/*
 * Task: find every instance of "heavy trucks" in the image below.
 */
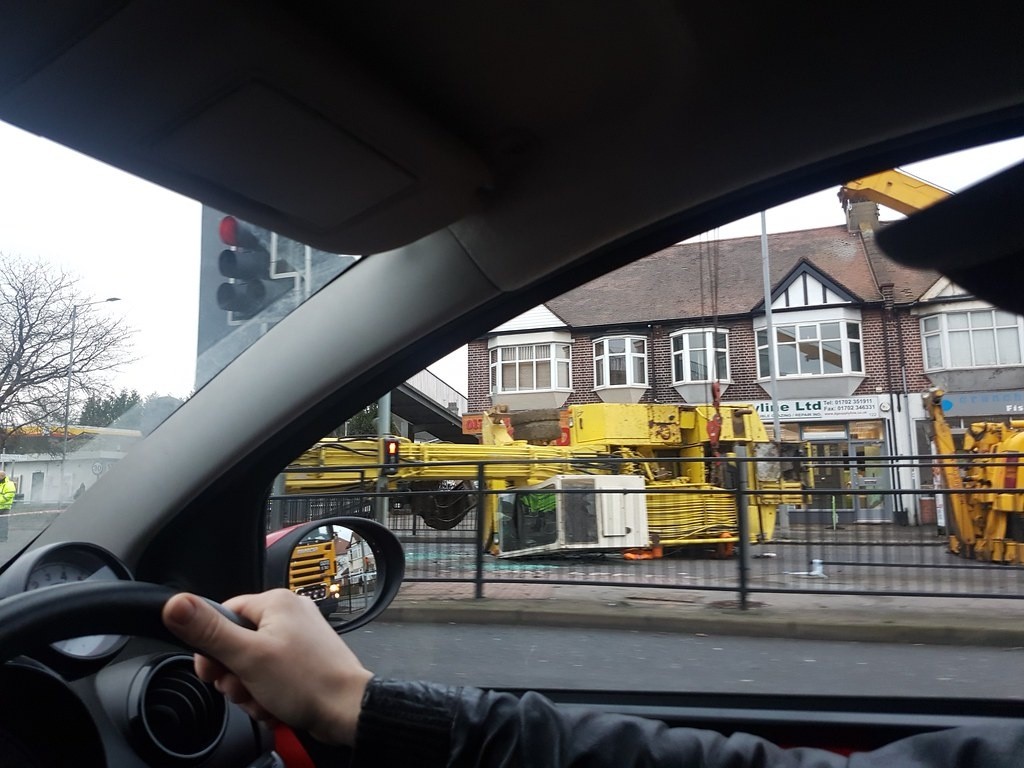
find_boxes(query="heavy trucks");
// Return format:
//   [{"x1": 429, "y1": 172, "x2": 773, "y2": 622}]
[{"x1": 287, "y1": 524, "x2": 344, "y2": 617}]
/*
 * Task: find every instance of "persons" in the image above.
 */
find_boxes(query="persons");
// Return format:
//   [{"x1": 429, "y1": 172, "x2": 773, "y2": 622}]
[
  {"x1": 0, "y1": 471, "x2": 16, "y2": 542},
  {"x1": 162, "y1": 585, "x2": 1022, "y2": 768},
  {"x1": 72, "y1": 482, "x2": 86, "y2": 500}
]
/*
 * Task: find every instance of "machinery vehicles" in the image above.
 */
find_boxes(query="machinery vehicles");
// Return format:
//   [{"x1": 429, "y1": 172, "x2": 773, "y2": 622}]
[
  {"x1": 283, "y1": 389, "x2": 782, "y2": 553},
  {"x1": 836, "y1": 160, "x2": 1023, "y2": 565}
]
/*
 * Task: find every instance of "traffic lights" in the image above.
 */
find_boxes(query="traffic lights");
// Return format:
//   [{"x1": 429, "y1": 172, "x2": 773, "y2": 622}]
[{"x1": 215, "y1": 209, "x2": 286, "y2": 324}]
